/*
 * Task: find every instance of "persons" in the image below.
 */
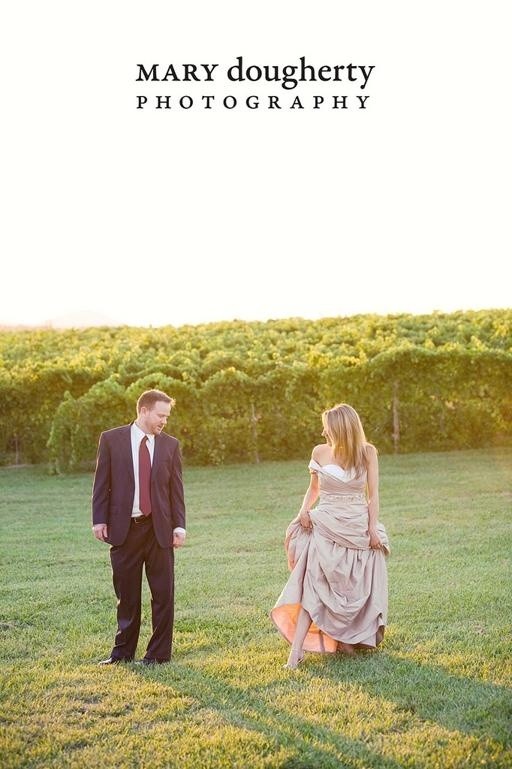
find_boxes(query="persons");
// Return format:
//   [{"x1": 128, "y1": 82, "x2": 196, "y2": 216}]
[
  {"x1": 265, "y1": 402, "x2": 391, "y2": 673},
  {"x1": 89, "y1": 389, "x2": 186, "y2": 667}
]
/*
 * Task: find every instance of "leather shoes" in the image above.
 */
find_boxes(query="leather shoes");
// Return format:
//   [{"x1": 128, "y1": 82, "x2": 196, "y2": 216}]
[
  {"x1": 99, "y1": 655, "x2": 132, "y2": 663},
  {"x1": 135, "y1": 657, "x2": 164, "y2": 665}
]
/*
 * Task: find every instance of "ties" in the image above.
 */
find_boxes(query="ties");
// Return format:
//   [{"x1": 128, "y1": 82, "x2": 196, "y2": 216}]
[{"x1": 139, "y1": 436, "x2": 152, "y2": 517}]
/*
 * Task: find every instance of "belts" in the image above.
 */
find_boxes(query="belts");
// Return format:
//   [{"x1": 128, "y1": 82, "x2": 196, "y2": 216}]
[{"x1": 132, "y1": 516, "x2": 148, "y2": 524}]
